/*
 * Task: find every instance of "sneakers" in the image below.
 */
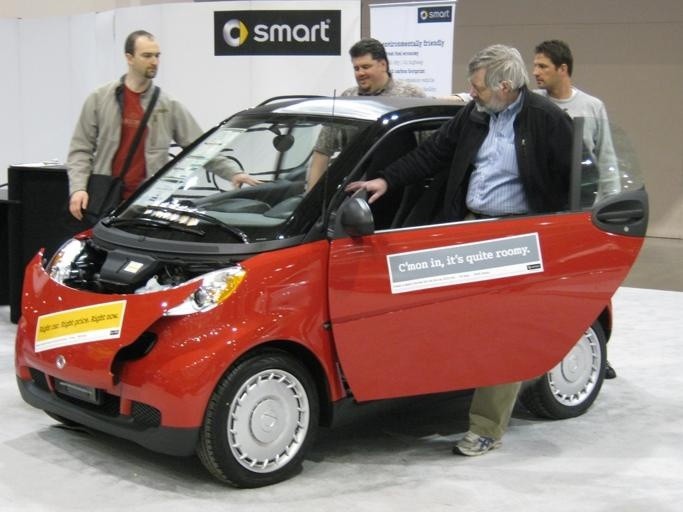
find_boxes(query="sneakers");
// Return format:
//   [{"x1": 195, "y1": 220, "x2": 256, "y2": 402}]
[{"x1": 452, "y1": 432, "x2": 502, "y2": 456}]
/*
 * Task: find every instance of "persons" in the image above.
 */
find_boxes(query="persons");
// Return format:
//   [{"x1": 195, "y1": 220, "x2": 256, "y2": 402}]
[
  {"x1": 306, "y1": 37, "x2": 435, "y2": 219},
  {"x1": 343, "y1": 44, "x2": 599, "y2": 458},
  {"x1": 429, "y1": 39, "x2": 621, "y2": 379},
  {"x1": 66, "y1": 29, "x2": 263, "y2": 232}
]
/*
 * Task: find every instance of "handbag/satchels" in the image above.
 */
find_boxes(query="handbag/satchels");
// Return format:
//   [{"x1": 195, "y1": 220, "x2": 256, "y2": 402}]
[{"x1": 61, "y1": 172, "x2": 124, "y2": 226}]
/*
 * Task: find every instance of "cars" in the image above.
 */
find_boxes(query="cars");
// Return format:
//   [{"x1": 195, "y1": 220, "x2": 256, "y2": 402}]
[{"x1": 16, "y1": 91, "x2": 648, "y2": 489}]
